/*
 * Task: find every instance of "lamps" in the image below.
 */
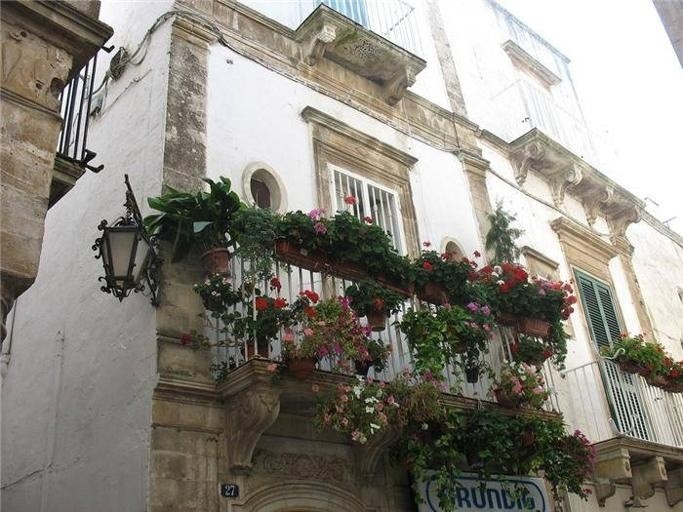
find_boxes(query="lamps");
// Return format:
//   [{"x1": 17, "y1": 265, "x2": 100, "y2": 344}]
[{"x1": 92, "y1": 174, "x2": 159, "y2": 308}]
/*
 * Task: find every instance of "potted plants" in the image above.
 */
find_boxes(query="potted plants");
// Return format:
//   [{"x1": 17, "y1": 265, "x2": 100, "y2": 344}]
[{"x1": 142, "y1": 176, "x2": 241, "y2": 281}]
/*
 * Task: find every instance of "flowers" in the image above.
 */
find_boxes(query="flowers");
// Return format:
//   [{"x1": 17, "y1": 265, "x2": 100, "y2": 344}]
[{"x1": 599, "y1": 330, "x2": 683, "y2": 394}]
